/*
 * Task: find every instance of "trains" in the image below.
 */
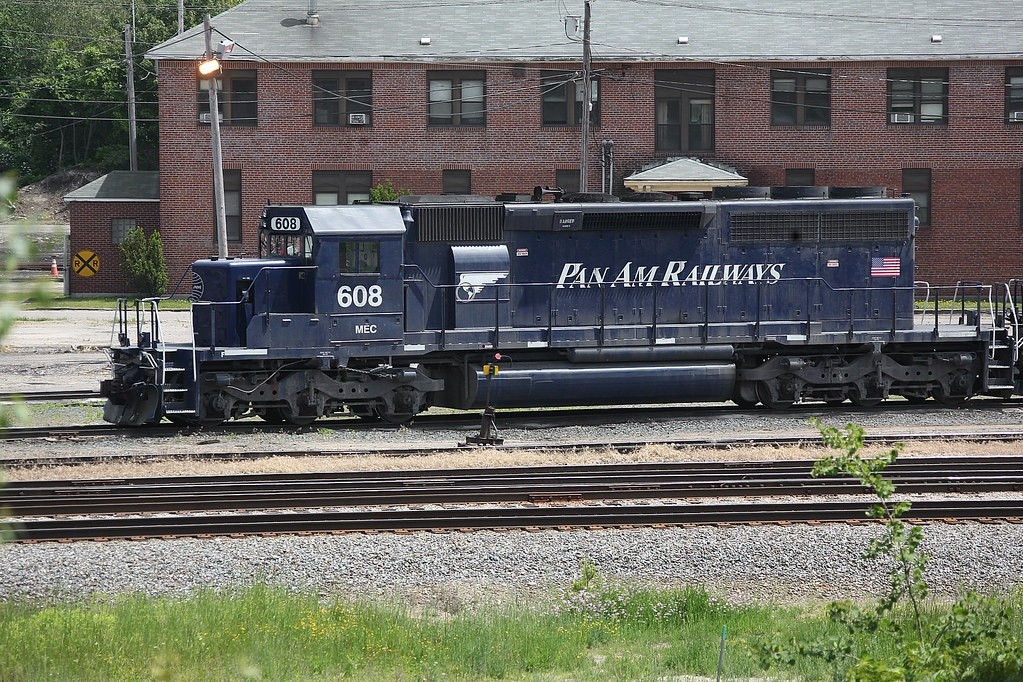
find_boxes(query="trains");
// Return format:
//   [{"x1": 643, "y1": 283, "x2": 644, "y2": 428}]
[{"x1": 100, "y1": 186, "x2": 1023, "y2": 429}]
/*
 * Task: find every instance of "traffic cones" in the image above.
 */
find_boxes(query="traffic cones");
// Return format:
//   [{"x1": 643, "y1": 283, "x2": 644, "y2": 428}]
[{"x1": 50, "y1": 258, "x2": 58, "y2": 275}]
